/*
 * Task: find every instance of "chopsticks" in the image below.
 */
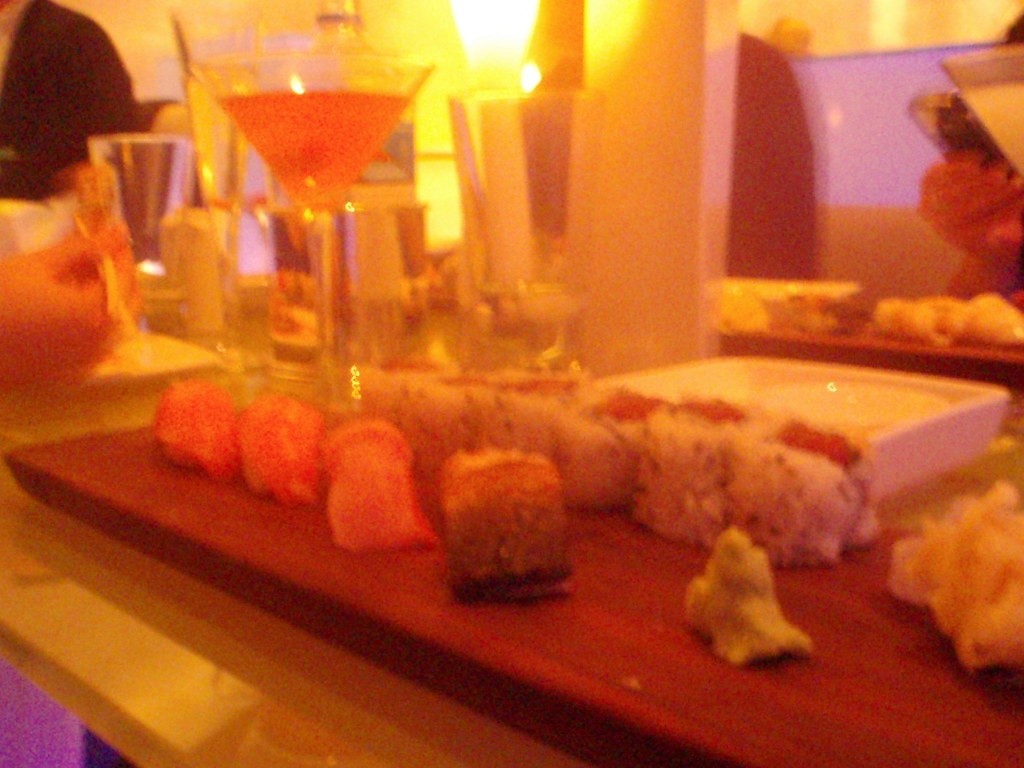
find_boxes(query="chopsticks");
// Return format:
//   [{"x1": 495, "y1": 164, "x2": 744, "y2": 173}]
[{"x1": 71, "y1": 210, "x2": 137, "y2": 338}]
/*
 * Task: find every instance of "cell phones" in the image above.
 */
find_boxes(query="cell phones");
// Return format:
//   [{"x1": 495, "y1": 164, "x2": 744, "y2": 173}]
[{"x1": 910, "y1": 91, "x2": 1018, "y2": 181}]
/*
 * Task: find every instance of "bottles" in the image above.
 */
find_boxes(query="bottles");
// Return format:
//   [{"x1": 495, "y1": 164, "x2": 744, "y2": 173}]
[{"x1": 264, "y1": 0, "x2": 429, "y2": 396}]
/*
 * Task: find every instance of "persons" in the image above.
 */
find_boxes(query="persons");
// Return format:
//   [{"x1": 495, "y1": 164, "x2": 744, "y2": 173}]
[
  {"x1": 1, "y1": 0, "x2": 145, "y2": 208},
  {"x1": 0, "y1": 165, "x2": 146, "y2": 401},
  {"x1": 917, "y1": 12, "x2": 1024, "y2": 310}
]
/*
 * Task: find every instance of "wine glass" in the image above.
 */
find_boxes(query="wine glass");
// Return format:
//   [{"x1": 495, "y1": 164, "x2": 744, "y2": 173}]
[{"x1": 189, "y1": 60, "x2": 437, "y2": 416}]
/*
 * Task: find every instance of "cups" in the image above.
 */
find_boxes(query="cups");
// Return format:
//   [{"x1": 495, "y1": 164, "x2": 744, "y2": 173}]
[
  {"x1": 450, "y1": 89, "x2": 603, "y2": 376},
  {"x1": 934, "y1": 43, "x2": 1024, "y2": 177},
  {"x1": 85, "y1": 133, "x2": 195, "y2": 347}
]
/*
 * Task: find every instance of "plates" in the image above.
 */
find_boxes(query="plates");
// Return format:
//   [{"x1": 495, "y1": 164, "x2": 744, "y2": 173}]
[{"x1": 587, "y1": 356, "x2": 1010, "y2": 493}]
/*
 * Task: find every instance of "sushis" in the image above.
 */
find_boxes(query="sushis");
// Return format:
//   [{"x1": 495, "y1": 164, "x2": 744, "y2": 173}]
[{"x1": 155, "y1": 363, "x2": 866, "y2": 591}]
